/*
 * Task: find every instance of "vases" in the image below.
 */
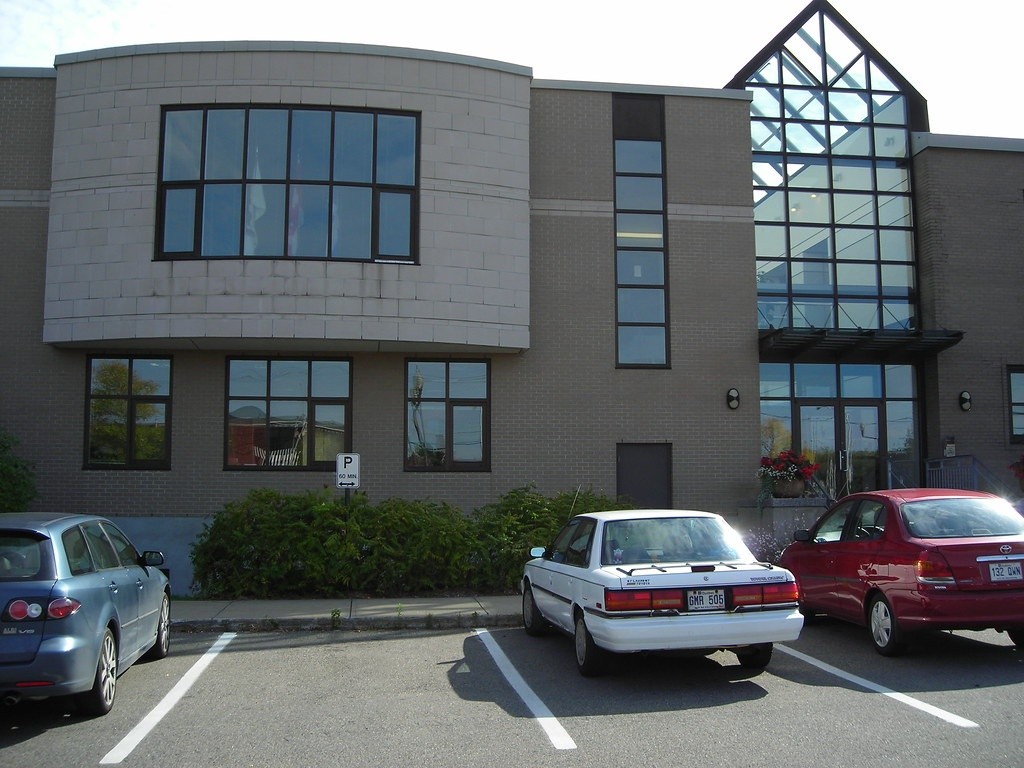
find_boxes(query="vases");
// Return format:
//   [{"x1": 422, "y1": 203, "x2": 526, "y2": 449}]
[{"x1": 772, "y1": 479, "x2": 804, "y2": 499}]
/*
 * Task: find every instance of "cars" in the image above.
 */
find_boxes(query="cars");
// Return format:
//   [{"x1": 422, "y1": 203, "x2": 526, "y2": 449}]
[
  {"x1": 776, "y1": 487, "x2": 1024, "y2": 657},
  {"x1": 0, "y1": 512, "x2": 172, "y2": 716},
  {"x1": 520, "y1": 508, "x2": 805, "y2": 677}
]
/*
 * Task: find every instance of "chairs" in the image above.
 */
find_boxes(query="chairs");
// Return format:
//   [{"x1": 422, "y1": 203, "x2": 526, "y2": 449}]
[
  {"x1": 873, "y1": 508, "x2": 884, "y2": 525},
  {"x1": 620, "y1": 544, "x2": 653, "y2": 563}
]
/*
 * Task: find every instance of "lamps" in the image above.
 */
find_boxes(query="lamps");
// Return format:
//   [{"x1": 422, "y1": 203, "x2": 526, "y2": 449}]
[
  {"x1": 727, "y1": 387, "x2": 739, "y2": 409},
  {"x1": 959, "y1": 391, "x2": 971, "y2": 411}
]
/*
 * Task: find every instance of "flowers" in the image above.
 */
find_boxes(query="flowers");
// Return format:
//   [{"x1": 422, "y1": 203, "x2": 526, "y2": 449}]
[{"x1": 754, "y1": 448, "x2": 820, "y2": 513}]
